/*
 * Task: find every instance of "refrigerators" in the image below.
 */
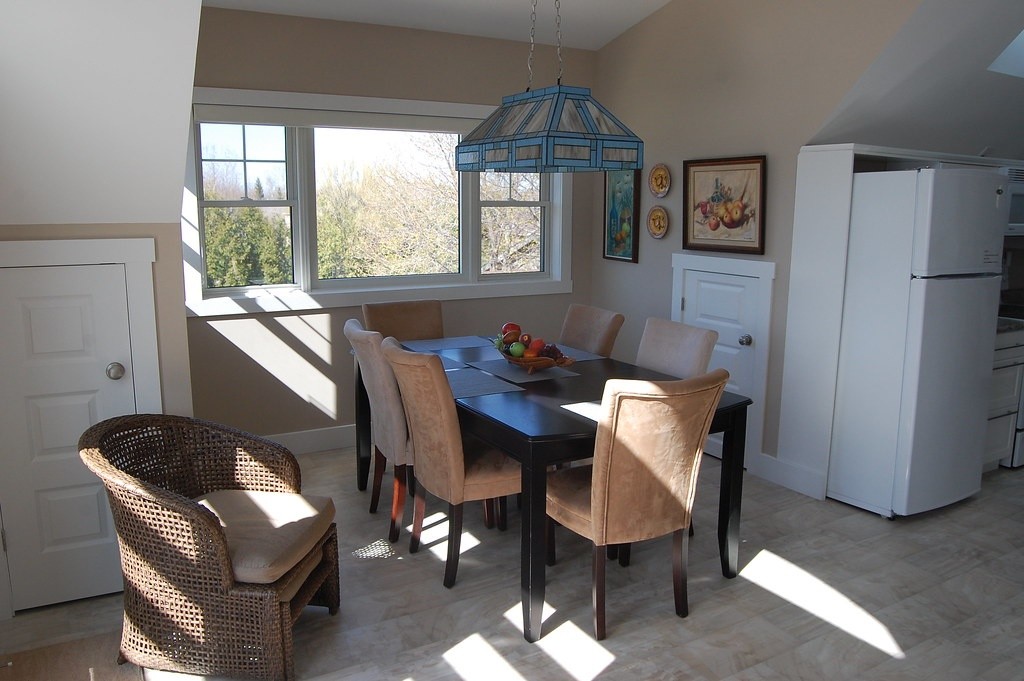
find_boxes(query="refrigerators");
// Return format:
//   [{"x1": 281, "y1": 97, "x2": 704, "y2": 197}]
[{"x1": 826, "y1": 164, "x2": 1010, "y2": 519}]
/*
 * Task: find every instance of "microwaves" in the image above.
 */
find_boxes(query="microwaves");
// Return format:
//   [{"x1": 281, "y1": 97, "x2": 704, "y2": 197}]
[{"x1": 998, "y1": 164, "x2": 1024, "y2": 238}]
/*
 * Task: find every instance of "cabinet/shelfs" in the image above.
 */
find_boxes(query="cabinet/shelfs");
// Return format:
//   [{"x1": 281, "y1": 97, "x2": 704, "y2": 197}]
[{"x1": 982, "y1": 342, "x2": 1024, "y2": 474}]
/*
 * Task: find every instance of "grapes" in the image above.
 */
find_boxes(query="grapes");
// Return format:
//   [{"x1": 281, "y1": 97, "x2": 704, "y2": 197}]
[
  {"x1": 503, "y1": 341, "x2": 513, "y2": 356},
  {"x1": 538, "y1": 343, "x2": 564, "y2": 361}
]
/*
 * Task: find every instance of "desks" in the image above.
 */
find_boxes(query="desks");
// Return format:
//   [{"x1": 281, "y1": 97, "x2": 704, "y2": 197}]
[{"x1": 398, "y1": 334, "x2": 754, "y2": 644}]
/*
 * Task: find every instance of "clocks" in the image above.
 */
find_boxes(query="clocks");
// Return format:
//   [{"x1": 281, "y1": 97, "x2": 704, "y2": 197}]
[
  {"x1": 646, "y1": 205, "x2": 670, "y2": 240},
  {"x1": 648, "y1": 163, "x2": 671, "y2": 199}
]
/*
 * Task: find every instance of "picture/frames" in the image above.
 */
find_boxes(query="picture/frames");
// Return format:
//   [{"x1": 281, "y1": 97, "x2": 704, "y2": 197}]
[
  {"x1": 602, "y1": 168, "x2": 641, "y2": 264},
  {"x1": 681, "y1": 155, "x2": 767, "y2": 255}
]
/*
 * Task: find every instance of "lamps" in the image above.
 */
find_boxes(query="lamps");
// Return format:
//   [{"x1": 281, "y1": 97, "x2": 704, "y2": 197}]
[{"x1": 453, "y1": 0, "x2": 650, "y2": 174}]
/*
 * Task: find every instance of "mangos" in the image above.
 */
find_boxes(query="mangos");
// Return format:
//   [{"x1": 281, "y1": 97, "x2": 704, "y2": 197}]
[{"x1": 501, "y1": 322, "x2": 545, "y2": 359}]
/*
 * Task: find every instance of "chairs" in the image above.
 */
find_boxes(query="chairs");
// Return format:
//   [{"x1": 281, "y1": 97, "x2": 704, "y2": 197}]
[
  {"x1": 635, "y1": 316, "x2": 720, "y2": 383},
  {"x1": 343, "y1": 318, "x2": 556, "y2": 589},
  {"x1": 544, "y1": 367, "x2": 730, "y2": 638},
  {"x1": 361, "y1": 300, "x2": 445, "y2": 340},
  {"x1": 74, "y1": 411, "x2": 343, "y2": 681},
  {"x1": 556, "y1": 303, "x2": 627, "y2": 359}
]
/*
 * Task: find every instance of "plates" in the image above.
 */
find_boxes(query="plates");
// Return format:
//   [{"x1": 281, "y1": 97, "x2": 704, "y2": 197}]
[
  {"x1": 648, "y1": 163, "x2": 671, "y2": 198},
  {"x1": 646, "y1": 205, "x2": 670, "y2": 240}
]
